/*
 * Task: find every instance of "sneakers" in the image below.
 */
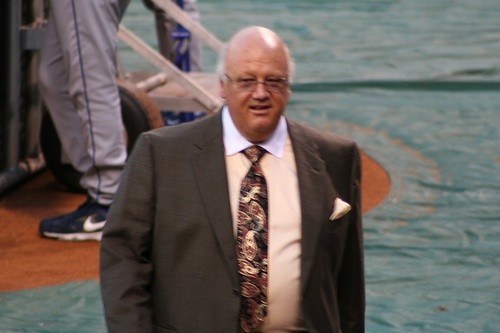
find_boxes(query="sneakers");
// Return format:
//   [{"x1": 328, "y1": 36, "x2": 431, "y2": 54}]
[{"x1": 38, "y1": 197, "x2": 110, "y2": 242}]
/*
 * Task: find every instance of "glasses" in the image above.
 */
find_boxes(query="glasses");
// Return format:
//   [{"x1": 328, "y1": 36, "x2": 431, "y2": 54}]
[{"x1": 224, "y1": 73, "x2": 286, "y2": 88}]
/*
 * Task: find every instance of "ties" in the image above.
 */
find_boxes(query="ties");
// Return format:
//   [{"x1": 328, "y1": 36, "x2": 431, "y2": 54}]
[{"x1": 236, "y1": 146, "x2": 268, "y2": 331}]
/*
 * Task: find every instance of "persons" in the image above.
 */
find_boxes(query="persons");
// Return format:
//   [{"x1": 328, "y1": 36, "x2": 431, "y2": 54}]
[
  {"x1": 35, "y1": 0, "x2": 129, "y2": 240},
  {"x1": 97, "y1": 25, "x2": 366, "y2": 333},
  {"x1": 143, "y1": 0, "x2": 213, "y2": 124}
]
